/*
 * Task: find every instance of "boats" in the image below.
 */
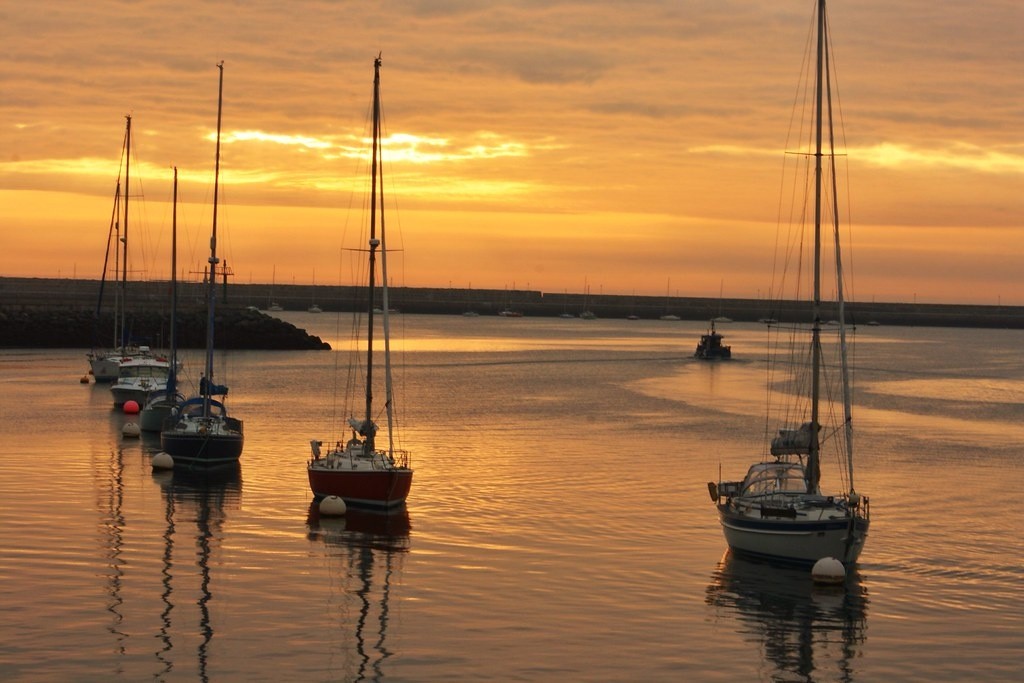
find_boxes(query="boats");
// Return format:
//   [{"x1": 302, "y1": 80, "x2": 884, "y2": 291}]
[{"x1": 694, "y1": 317, "x2": 731, "y2": 359}]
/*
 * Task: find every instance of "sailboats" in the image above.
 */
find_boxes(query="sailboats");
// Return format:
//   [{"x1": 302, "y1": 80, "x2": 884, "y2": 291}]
[
  {"x1": 709, "y1": 0, "x2": 868, "y2": 568},
  {"x1": 160, "y1": 60, "x2": 245, "y2": 478},
  {"x1": 268, "y1": 263, "x2": 282, "y2": 311},
  {"x1": 711, "y1": 278, "x2": 733, "y2": 323},
  {"x1": 308, "y1": 497, "x2": 411, "y2": 679},
  {"x1": 86, "y1": 115, "x2": 184, "y2": 432},
  {"x1": 462, "y1": 283, "x2": 479, "y2": 316},
  {"x1": 758, "y1": 289, "x2": 780, "y2": 323},
  {"x1": 308, "y1": 53, "x2": 413, "y2": 509},
  {"x1": 704, "y1": 544, "x2": 866, "y2": 683},
  {"x1": 661, "y1": 277, "x2": 681, "y2": 320},
  {"x1": 561, "y1": 281, "x2": 597, "y2": 319},
  {"x1": 499, "y1": 282, "x2": 521, "y2": 316},
  {"x1": 373, "y1": 276, "x2": 399, "y2": 314}
]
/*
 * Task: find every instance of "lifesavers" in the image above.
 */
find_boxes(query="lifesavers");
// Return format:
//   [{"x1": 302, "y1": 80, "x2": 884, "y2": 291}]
[
  {"x1": 707, "y1": 481, "x2": 718, "y2": 501},
  {"x1": 346, "y1": 439, "x2": 363, "y2": 449}
]
